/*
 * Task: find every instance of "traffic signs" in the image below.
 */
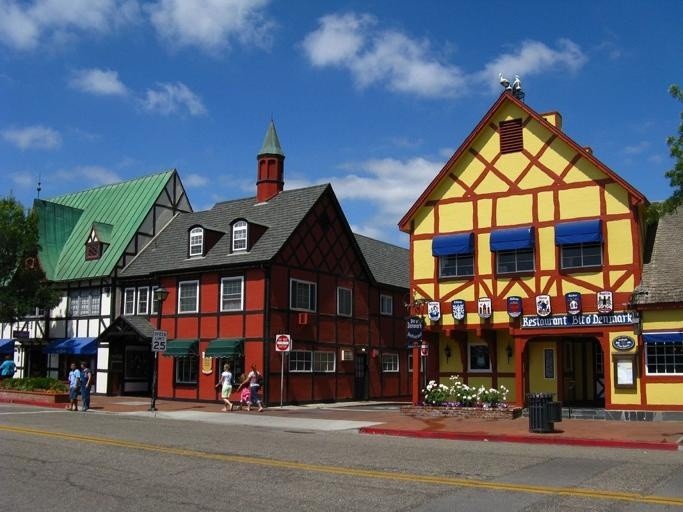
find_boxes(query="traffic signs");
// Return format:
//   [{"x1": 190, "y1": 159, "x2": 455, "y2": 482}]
[
  {"x1": 13, "y1": 331, "x2": 29, "y2": 340},
  {"x1": 150, "y1": 330, "x2": 166, "y2": 352}
]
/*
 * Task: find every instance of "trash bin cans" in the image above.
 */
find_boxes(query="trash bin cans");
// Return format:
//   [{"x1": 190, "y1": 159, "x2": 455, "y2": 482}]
[{"x1": 527, "y1": 392, "x2": 562, "y2": 433}]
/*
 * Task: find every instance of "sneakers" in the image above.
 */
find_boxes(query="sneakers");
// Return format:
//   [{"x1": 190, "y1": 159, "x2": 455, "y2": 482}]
[
  {"x1": 221, "y1": 403, "x2": 265, "y2": 412},
  {"x1": 66, "y1": 407, "x2": 90, "y2": 412}
]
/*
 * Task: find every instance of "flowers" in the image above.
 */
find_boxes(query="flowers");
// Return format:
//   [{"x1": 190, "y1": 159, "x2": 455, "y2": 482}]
[{"x1": 421, "y1": 373, "x2": 509, "y2": 407}]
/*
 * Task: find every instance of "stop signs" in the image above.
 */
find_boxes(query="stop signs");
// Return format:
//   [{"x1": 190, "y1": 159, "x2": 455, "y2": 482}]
[{"x1": 274, "y1": 334, "x2": 290, "y2": 352}]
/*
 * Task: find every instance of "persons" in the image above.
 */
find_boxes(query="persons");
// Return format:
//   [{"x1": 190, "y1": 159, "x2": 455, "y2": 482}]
[
  {"x1": 241, "y1": 362, "x2": 264, "y2": 412},
  {"x1": 215, "y1": 364, "x2": 234, "y2": 412},
  {"x1": 66, "y1": 363, "x2": 81, "y2": 411},
  {"x1": 0, "y1": 355, "x2": 17, "y2": 378},
  {"x1": 237, "y1": 374, "x2": 253, "y2": 411},
  {"x1": 78, "y1": 361, "x2": 93, "y2": 411}
]
